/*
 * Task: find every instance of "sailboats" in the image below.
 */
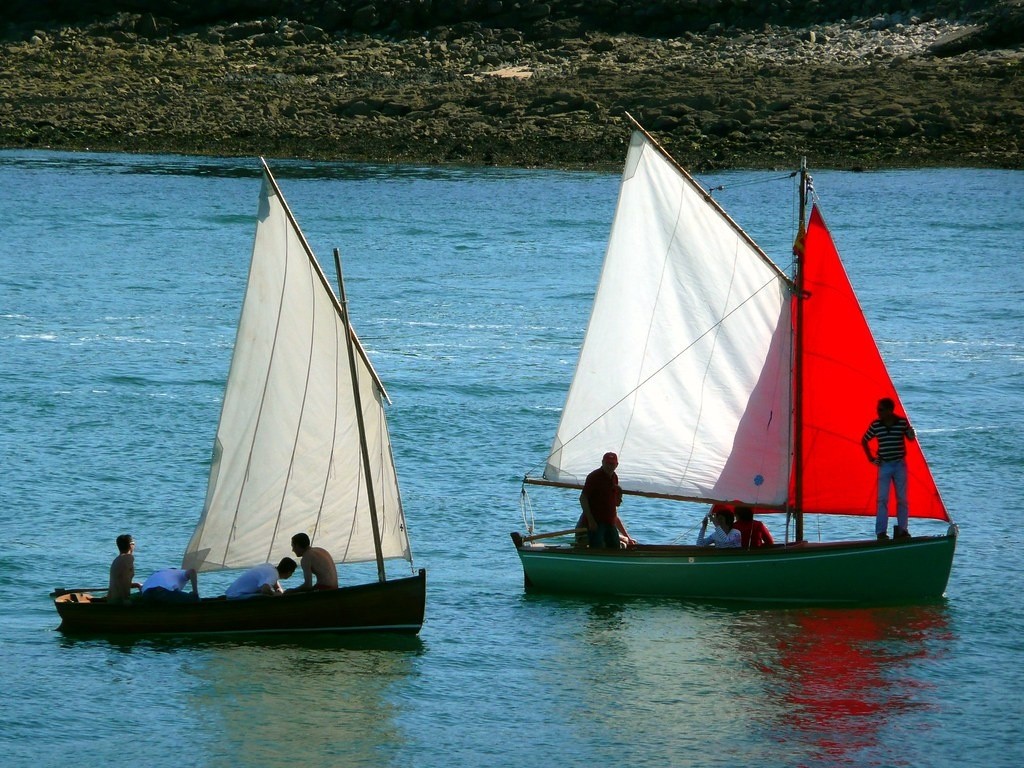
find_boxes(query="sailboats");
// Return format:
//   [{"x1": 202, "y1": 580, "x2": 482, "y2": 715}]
[
  {"x1": 51, "y1": 147, "x2": 428, "y2": 642},
  {"x1": 510, "y1": 106, "x2": 959, "y2": 613}
]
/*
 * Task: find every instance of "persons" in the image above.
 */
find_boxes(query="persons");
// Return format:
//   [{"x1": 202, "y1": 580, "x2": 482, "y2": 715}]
[
  {"x1": 697, "y1": 507, "x2": 774, "y2": 548},
  {"x1": 225, "y1": 557, "x2": 298, "y2": 600},
  {"x1": 861, "y1": 398, "x2": 916, "y2": 542},
  {"x1": 284, "y1": 533, "x2": 338, "y2": 593},
  {"x1": 107, "y1": 535, "x2": 201, "y2": 604},
  {"x1": 575, "y1": 452, "x2": 636, "y2": 550}
]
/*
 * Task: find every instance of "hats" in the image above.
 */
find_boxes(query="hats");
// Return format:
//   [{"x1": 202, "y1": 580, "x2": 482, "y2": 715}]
[{"x1": 603, "y1": 452, "x2": 618, "y2": 465}]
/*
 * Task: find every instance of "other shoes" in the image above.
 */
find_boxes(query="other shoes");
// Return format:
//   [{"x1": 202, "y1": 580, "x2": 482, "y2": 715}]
[{"x1": 877, "y1": 532, "x2": 890, "y2": 540}]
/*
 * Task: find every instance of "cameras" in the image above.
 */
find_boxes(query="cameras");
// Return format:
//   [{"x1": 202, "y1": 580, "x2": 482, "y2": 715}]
[{"x1": 708, "y1": 514, "x2": 715, "y2": 521}]
